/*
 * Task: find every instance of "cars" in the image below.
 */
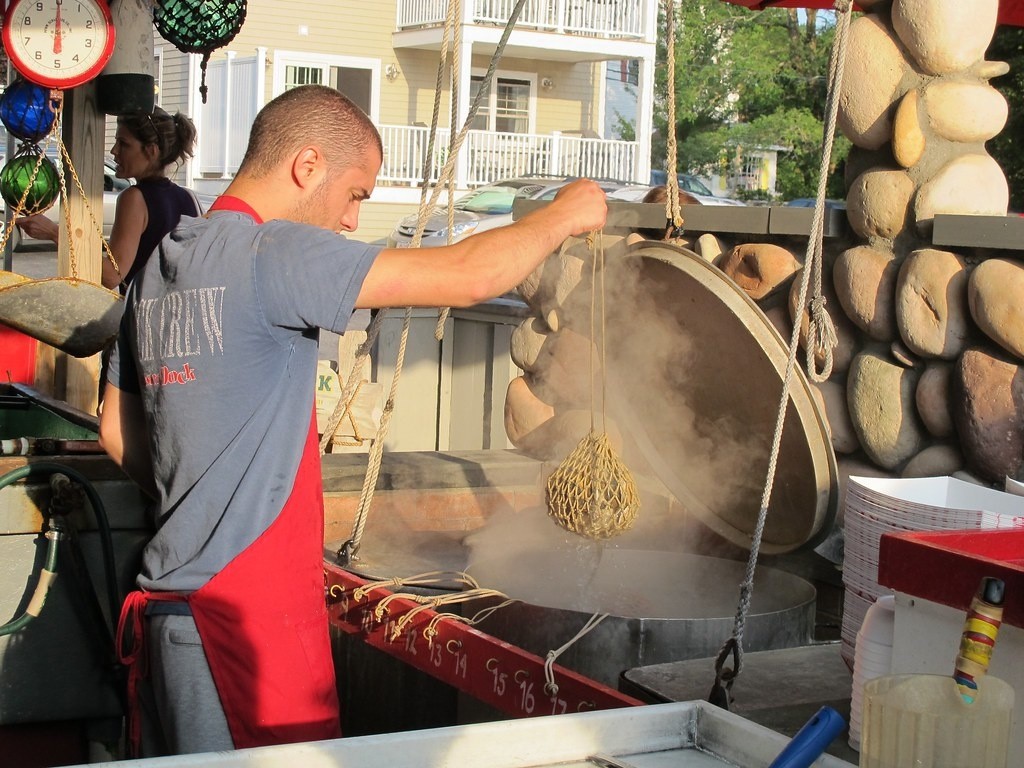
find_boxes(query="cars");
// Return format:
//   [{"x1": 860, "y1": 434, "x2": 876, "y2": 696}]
[
  {"x1": 387, "y1": 170, "x2": 847, "y2": 248},
  {"x1": 0, "y1": 147, "x2": 219, "y2": 252}
]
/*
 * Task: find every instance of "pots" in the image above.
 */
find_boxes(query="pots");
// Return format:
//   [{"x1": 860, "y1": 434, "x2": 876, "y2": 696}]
[
  {"x1": 458, "y1": 548, "x2": 817, "y2": 726},
  {"x1": 322, "y1": 531, "x2": 459, "y2": 737}
]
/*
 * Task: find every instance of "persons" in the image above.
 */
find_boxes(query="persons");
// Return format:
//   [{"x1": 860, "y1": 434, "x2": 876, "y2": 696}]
[
  {"x1": 97, "y1": 83, "x2": 608, "y2": 758},
  {"x1": 640, "y1": 186, "x2": 705, "y2": 241},
  {"x1": 13, "y1": 104, "x2": 205, "y2": 415}
]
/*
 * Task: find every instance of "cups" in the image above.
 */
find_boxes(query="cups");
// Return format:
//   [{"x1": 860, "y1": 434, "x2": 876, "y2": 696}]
[{"x1": 858, "y1": 673, "x2": 1014, "y2": 768}]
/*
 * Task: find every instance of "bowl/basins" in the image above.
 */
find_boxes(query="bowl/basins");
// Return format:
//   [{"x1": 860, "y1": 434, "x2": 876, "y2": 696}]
[{"x1": 848, "y1": 594, "x2": 896, "y2": 753}]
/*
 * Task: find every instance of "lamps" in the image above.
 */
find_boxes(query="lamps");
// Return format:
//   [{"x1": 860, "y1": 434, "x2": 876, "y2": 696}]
[
  {"x1": 541, "y1": 77, "x2": 555, "y2": 92},
  {"x1": 385, "y1": 63, "x2": 400, "y2": 82}
]
